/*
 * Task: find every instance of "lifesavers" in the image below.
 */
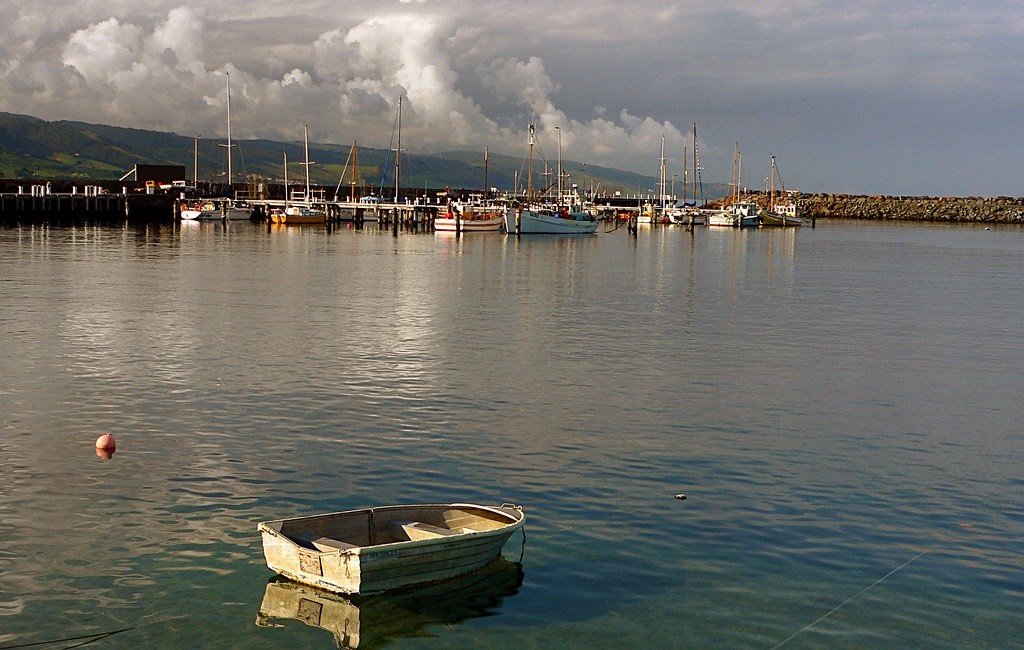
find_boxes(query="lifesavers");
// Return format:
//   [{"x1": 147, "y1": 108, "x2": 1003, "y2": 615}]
[
  {"x1": 195, "y1": 204, "x2": 201, "y2": 212},
  {"x1": 180, "y1": 203, "x2": 187, "y2": 212}
]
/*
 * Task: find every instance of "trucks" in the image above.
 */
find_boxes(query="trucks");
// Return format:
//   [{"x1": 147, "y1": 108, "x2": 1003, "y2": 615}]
[
  {"x1": 134, "y1": 181, "x2": 160, "y2": 193},
  {"x1": 158, "y1": 181, "x2": 186, "y2": 193}
]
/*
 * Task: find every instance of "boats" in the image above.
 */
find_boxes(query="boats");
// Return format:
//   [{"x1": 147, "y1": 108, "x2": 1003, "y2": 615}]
[
  {"x1": 494, "y1": 166, "x2": 678, "y2": 206},
  {"x1": 433, "y1": 186, "x2": 505, "y2": 232},
  {"x1": 638, "y1": 193, "x2": 652, "y2": 223},
  {"x1": 256, "y1": 504, "x2": 526, "y2": 597},
  {"x1": 253, "y1": 558, "x2": 526, "y2": 650}
]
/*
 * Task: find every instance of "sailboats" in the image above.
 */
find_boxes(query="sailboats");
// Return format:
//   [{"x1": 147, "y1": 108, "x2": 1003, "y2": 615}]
[
  {"x1": 667, "y1": 125, "x2": 709, "y2": 225},
  {"x1": 710, "y1": 142, "x2": 763, "y2": 226},
  {"x1": 178, "y1": 71, "x2": 265, "y2": 221},
  {"x1": 269, "y1": 124, "x2": 326, "y2": 223},
  {"x1": 503, "y1": 109, "x2": 600, "y2": 235},
  {"x1": 652, "y1": 134, "x2": 672, "y2": 223},
  {"x1": 762, "y1": 154, "x2": 803, "y2": 226},
  {"x1": 326, "y1": 140, "x2": 395, "y2": 221},
  {"x1": 375, "y1": 95, "x2": 433, "y2": 222}
]
